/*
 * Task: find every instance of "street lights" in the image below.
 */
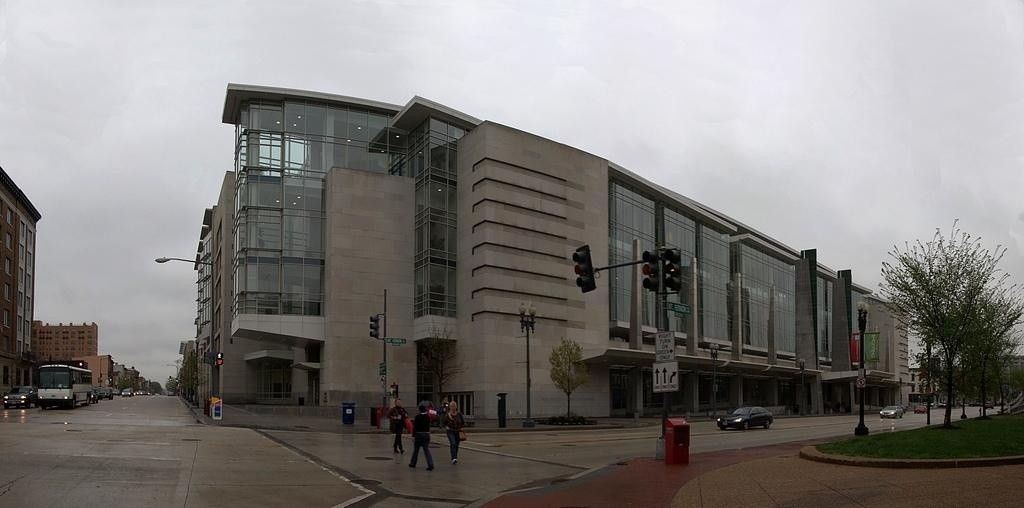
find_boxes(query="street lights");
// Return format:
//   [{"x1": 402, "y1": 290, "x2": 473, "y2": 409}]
[
  {"x1": 517, "y1": 301, "x2": 536, "y2": 428},
  {"x1": 855, "y1": 299, "x2": 869, "y2": 435},
  {"x1": 155, "y1": 257, "x2": 218, "y2": 399},
  {"x1": 798, "y1": 359, "x2": 807, "y2": 416},
  {"x1": 165, "y1": 364, "x2": 179, "y2": 396},
  {"x1": 709, "y1": 343, "x2": 719, "y2": 420}
]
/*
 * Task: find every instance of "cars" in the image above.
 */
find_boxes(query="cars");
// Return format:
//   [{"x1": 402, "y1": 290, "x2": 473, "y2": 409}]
[
  {"x1": 879, "y1": 406, "x2": 903, "y2": 418},
  {"x1": 717, "y1": 406, "x2": 773, "y2": 431},
  {"x1": 914, "y1": 398, "x2": 993, "y2": 414},
  {"x1": 91, "y1": 387, "x2": 160, "y2": 404}
]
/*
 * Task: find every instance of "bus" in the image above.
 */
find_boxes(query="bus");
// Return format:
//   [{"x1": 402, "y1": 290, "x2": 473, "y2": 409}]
[{"x1": 37, "y1": 364, "x2": 91, "y2": 409}]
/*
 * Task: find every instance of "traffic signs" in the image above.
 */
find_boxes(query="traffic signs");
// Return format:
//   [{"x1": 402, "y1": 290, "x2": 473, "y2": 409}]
[
  {"x1": 384, "y1": 337, "x2": 406, "y2": 344},
  {"x1": 652, "y1": 363, "x2": 678, "y2": 392},
  {"x1": 384, "y1": 392, "x2": 396, "y2": 396},
  {"x1": 667, "y1": 303, "x2": 690, "y2": 313},
  {"x1": 654, "y1": 331, "x2": 674, "y2": 361},
  {"x1": 379, "y1": 362, "x2": 385, "y2": 375}
]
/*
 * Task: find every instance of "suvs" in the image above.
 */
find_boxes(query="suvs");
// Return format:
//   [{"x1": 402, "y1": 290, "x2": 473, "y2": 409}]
[{"x1": 3, "y1": 385, "x2": 38, "y2": 409}]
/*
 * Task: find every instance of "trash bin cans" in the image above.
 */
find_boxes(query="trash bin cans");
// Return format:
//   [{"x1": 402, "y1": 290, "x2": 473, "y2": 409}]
[
  {"x1": 665, "y1": 419, "x2": 689, "y2": 464},
  {"x1": 342, "y1": 402, "x2": 355, "y2": 425},
  {"x1": 370, "y1": 406, "x2": 377, "y2": 426},
  {"x1": 376, "y1": 406, "x2": 386, "y2": 428}
]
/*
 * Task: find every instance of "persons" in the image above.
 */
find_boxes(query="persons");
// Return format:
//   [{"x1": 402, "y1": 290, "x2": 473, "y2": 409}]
[
  {"x1": 444, "y1": 400, "x2": 468, "y2": 464},
  {"x1": 385, "y1": 396, "x2": 406, "y2": 451},
  {"x1": 438, "y1": 400, "x2": 447, "y2": 428},
  {"x1": 408, "y1": 400, "x2": 434, "y2": 470}
]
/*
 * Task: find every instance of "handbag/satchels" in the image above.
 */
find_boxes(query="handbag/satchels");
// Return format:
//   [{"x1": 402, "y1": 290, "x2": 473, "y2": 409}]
[
  {"x1": 404, "y1": 418, "x2": 414, "y2": 433},
  {"x1": 459, "y1": 430, "x2": 466, "y2": 441}
]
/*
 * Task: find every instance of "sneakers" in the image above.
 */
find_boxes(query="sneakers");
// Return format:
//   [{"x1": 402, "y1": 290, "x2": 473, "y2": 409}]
[{"x1": 452, "y1": 459, "x2": 457, "y2": 464}]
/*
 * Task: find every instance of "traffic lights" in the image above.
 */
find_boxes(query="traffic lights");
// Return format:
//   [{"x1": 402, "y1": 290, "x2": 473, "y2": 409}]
[
  {"x1": 169, "y1": 375, "x2": 171, "y2": 381},
  {"x1": 369, "y1": 315, "x2": 378, "y2": 339},
  {"x1": 642, "y1": 248, "x2": 659, "y2": 293},
  {"x1": 572, "y1": 245, "x2": 595, "y2": 293},
  {"x1": 215, "y1": 352, "x2": 223, "y2": 366},
  {"x1": 663, "y1": 246, "x2": 680, "y2": 293},
  {"x1": 203, "y1": 352, "x2": 212, "y2": 365}
]
